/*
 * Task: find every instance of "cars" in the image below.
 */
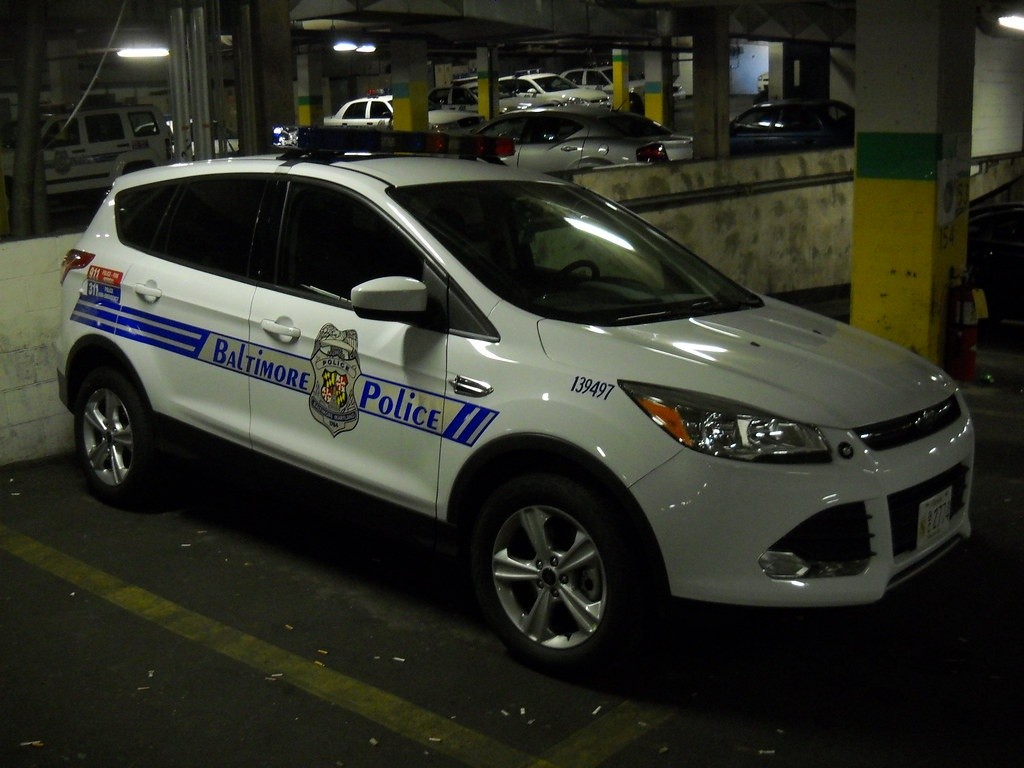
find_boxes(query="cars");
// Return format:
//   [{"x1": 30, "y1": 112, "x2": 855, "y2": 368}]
[
  {"x1": 54, "y1": 121, "x2": 974, "y2": 684},
  {"x1": 497, "y1": 69, "x2": 612, "y2": 114},
  {"x1": 727, "y1": 97, "x2": 856, "y2": 157},
  {"x1": 562, "y1": 65, "x2": 689, "y2": 110},
  {"x1": 470, "y1": 105, "x2": 695, "y2": 177},
  {"x1": 426, "y1": 76, "x2": 545, "y2": 114},
  {"x1": 943, "y1": 201, "x2": 1024, "y2": 446},
  {"x1": 319, "y1": 88, "x2": 485, "y2": 131},
  {"x1": 756, "y1": 71, "x2": 770, "y2": 94}
]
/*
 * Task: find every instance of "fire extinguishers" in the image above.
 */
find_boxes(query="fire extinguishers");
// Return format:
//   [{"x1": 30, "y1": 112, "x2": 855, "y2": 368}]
[{"x1": 942, "y1": 275, "x2": 980, "y2": 385}]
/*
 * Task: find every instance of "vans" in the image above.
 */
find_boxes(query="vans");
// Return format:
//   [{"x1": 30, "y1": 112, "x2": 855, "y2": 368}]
[{"x1": 2, "y1": 105, "x2": 173, "y2": 220}]
